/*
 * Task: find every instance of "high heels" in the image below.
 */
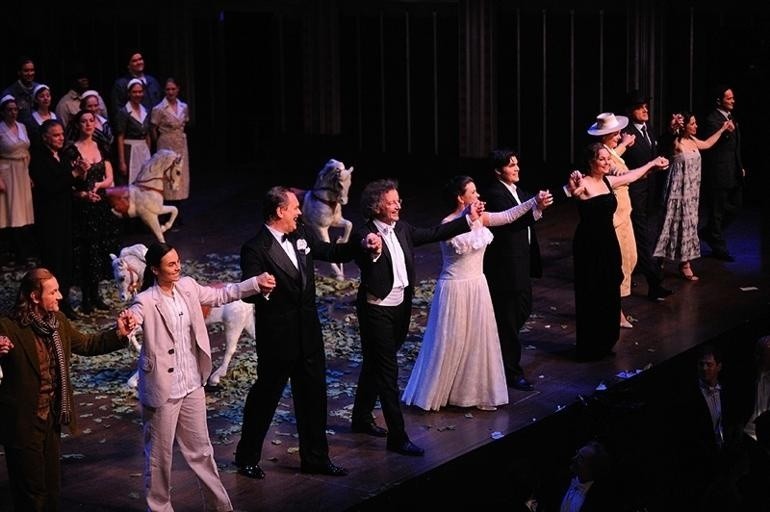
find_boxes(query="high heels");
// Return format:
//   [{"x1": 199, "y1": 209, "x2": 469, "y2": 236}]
[{"x1": 678, "y1": 261, "x2": 700, "y2": 281}]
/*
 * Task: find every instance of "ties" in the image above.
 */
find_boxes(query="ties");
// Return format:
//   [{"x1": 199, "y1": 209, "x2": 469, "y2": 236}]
[
  {"x1": 728, "y1": 115, "x2": 731, "y2": 121},
  {"x1": 641, "y1": 127, "x2": 649, "y2": 145}
]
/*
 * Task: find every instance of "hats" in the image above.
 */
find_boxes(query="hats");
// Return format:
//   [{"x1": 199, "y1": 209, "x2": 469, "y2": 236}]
[
  {"x1": 623, "y1": 88, "x2": 653, "y2": 112},
  {"x1": 80, "y1": 89, "x2": 99, "y2": 100},
  {"x1": 126, "y1": 78, "x2": 142, "y2": 89},
  {"x1": 30, "y1": 83, "x2": 50, "y2": 100},
  {"x1": 0, "y1": 94, "x2": 16, "y2": 105},
  {"x1": 587, "y1": 112, "x2": 628, "y2": 135}
]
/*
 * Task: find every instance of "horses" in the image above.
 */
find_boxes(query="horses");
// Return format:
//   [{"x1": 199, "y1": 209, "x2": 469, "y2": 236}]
[
  {"x1": 109, "y1": 242, "x2": 255, "y2": 391},
  {"x1": 288, "y1": 159, "x2": 354, "y2": 282},
  {"x1": 105, "y1": 148, "x2": 185, "y2": 252}
]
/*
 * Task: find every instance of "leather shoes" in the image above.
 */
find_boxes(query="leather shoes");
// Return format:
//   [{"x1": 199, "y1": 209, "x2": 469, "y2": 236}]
[
  {"x1": 647, "y1": 287, "x2": 674, "y2": 299},
  {"x1": 300, "y1": 461, "x2": 348, "y2": 477},
  {"x1": 351, "y1": 420, "x2": 387, "y2": 437},
  {"x1": 386, "y1": 435, "x2": 426, "y2": 457},
  {"x1": 506, "y1": 375, "x2": 534, "y2": 392},
  {"x1": 236, "y1": 464, "x2": 265, "y2": 479}
]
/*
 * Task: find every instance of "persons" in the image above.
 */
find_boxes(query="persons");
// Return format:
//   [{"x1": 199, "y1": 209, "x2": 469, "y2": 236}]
[
  {"x1": 653, "y1": 109, "x2": 733, "y2": 281},
  {"x1": 151, "y1": 79, "x2": 190, "y2": 226},
  {"x1": 555, "y1": 443, "x2": 604, "y2": 512},
  {"x1": 569, "y1": 143, "x2": 668, "y2": 362},
  {"x1": 28, "y1": 119, "x2": 90, "y2": 320},
  {"x1": 1, "y1": 268, "x2": 135, "y2": 511},
  {"x1": 0, "y1": 95, "x2": 34, "y2": 229},
  {"x1": 694, "y1": 345, "x2": 723, "y2": 444},
  {"x1": 483, "y1": 151, "x2": 543, "y2": 392},
  {"x1": 587, "y1": 111, "x2": 669, "y2": 329},
  {"x1": 118, "y1": 242, "x2": 276, "y2": 512},
  {"x1": 619, "y1": 88, "x2": 682, "y2": 300},
  {"x1": 64, "y1": 109, "x2": 121, "y2": 310},
  {"x1": 402, "y1": 175, "x2": 548, "y2": 412},
  {"x1": 0, "y1": 339, "x2": 8, "y2": 354},
  {"x1": 55, "y1": 74, "x2": 107, "y2": 126},
  {"x1": 64, "y1": 90, "x2": 113, "y2": 156},
  {"x1": 235, "y1": 186, "x2": 378, "y2": 480},
  {"x1": 705, "y1": 81, "x2": 745, "y2": 263},
  {"x1": 113, "y1": 79, "x2": 151, "y2": 188},
  {"x1": 3, "y1": 59, "x2": 40, "y2": 121},
  {"x1": 27, "y1": 85, "x2": 59, "y2": 138},
  {"x1": 350, "y1": 180, "x2": 484, "y2": 455},
  {"x1": 114, "y1": 53, "x2": 160, "y2": 115}
]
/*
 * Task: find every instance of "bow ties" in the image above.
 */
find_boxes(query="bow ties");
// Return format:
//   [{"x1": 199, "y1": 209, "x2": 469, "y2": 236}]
[
  {"x1": 281, "y1": 233, "x2": 292, "y2": 243},
  {"x1": 705, "y1": 384, "x2": 721, "y2": 393},
  {"x1": 570, "y1": 478, "x2": 585, "y2": 493}
]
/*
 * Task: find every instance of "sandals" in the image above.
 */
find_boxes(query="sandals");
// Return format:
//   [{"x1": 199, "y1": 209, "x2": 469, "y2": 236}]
[{"x1": 620, "y1": 320, "x2": 633, "y2": 328}]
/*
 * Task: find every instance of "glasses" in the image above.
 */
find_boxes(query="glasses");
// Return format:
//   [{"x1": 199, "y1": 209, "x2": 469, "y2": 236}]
[{"x1": 385, "y1": 199, "x2": 402, "y2": 207}]
